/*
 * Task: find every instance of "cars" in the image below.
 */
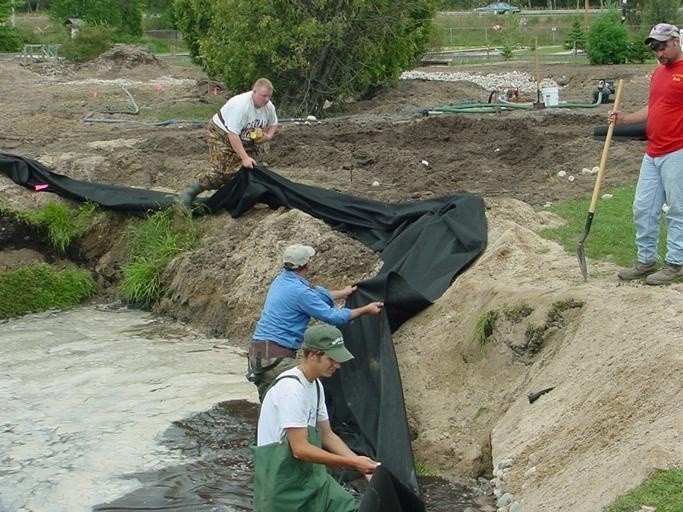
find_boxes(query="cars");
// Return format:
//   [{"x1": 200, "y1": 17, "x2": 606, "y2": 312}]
[{"x1": 474, "y1": 1, "x2": 520, "y2": 15}]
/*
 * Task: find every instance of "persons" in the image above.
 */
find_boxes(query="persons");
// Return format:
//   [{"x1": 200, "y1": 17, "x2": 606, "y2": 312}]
[
  {"x1": 247, "y1": 243, "x2": 385, "y2": 404},
  {"x1": 248, "y1": 322, "x2": 381, "y2": 512},
  {"x1": 606, "y1": 23, "x2": 683, "y2": 286},
  {"x1": 178, "y1": 77, "x2": 278, "y2": 214}
]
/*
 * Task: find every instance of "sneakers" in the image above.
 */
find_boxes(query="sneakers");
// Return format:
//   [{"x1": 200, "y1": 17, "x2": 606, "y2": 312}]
[{"x1": 617, "y1": 261, "x2": 683, "y2": 285}]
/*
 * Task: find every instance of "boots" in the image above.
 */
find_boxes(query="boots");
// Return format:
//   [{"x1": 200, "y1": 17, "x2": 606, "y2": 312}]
[{"x1": 181, "y1": 177, "x2": 204, "y2": 208}]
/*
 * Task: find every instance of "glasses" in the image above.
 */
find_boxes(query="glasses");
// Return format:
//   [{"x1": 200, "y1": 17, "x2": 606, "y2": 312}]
[{"x1": 650, "y1": 42, "x2": 668, "y2": 52}]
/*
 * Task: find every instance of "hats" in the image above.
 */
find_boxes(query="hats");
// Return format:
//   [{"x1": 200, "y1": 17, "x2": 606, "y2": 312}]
[
  {"x1": 283, "y1": 243, "x2": 316, "y2": 270},
  {"x1": 302, "y1": 323, "x2": 356, "y2": 363},
  {"x1": 644, "y1": 22, "x2": 681, "y2": 44}
]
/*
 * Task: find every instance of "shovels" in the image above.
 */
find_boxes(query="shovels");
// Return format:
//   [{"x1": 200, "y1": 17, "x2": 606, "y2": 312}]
[
  {"x1": 533, "y1": 37, "x2": 545, "y2": 109},
  {"x1": 577, "y1": 79, "x2": 623, "y2": 282}
]
